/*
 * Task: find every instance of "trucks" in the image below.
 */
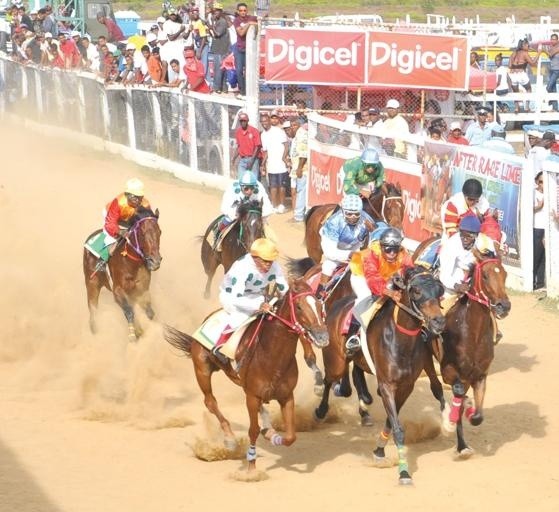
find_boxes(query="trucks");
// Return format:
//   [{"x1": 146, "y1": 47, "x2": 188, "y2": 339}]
[{"x1": 1, "y1": 0, "x2": 119, "y2": 51}]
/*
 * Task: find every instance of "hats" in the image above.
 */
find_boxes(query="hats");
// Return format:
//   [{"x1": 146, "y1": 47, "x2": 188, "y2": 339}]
[
  {"x1": 450, "y1": 121, "x2": 461, "y2": 131},
  {"x1": 184, "y1": 50, "x2": 193, "y2": 58},
  {"x1": 239, "y1": 113, "x2": 248, "y2": 121},
  {"x1": 368, "y1": 107, "x2": 380, "y2": 114},
  {"x1": 45, "y1": 32, "x2": 52, "y2": 38},
  {"x1": 386, "y1": 99, "x2": 400, "y2": 108},
  {"x1": 113, "y1": 50, "x2": 121, "y2": 56},
  {"x1": 126, "y1": 44, "x2": 135, "y2": 50},
  {"x1": 476, "y1": 107, "x2": 490, "y2": 115}
]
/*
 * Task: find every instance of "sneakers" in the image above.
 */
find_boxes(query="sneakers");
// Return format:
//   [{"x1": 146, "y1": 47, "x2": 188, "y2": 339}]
[{"x1": 276, "y1": 204, "x2": 285, "y2": 213}]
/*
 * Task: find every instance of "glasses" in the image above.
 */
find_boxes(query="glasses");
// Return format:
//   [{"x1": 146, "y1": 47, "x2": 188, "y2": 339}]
[
  {"x1": 384, "y1": 246, "x2": 399, "y2": 253},
  {"x1": 344, "y1": 210, "x2": 361, "y2": 218}
]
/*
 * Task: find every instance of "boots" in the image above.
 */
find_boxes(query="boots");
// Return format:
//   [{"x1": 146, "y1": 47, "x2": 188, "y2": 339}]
[
  {"x1": 211, "y1": 325, "x2": 234, "y2": 364},
  {"x1": 343, "y1": 315, "x2": 360, "y2": 357}
]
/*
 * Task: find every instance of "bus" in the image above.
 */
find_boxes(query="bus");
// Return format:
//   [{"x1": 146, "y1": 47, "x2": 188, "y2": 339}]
[{"x1": 471, "y1": 44, "x2": 552, "y2": 86}]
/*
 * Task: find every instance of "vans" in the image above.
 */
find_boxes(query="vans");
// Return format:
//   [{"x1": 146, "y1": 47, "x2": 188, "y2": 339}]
[{"x1": 314, "y1": 14, "x2": 384, "y2": 23}]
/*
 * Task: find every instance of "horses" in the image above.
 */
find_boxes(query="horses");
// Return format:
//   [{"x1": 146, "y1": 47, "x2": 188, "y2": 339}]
[
  {"x1": 424, "y1": 244, "x2": 510, "y2": 459},
  {"x1": 411, "y1": 207, "x2": 503, "y2": 263},
  {"x1": 164, "y1": 277, "x2": 331, "y2": 471},
  {"x1": 313, "y1": 264, "x2": 447, "y2": 486},
  {"x1": 301, "y1": 182, "x2": 405, "y2": 264},
  {"x1": 283, "y1": 254, "x2": 358, "y2": 398},
  {"x1": 191, "y1": 197, "x2": 264, "y2": 298},
  {"x1": 83, "y1": 207, "x2": 163, "y2": 343}
]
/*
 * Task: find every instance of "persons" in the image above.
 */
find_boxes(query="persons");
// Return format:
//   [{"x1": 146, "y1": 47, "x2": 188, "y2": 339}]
[
  {"x1": 436, "y1": 214, "x2": 498, "y2": 346},
  {"x1": 0, "y1": 1, "x2": 559, "y2": 291},
  {"x1": 316, "y1": 194, "x2": 377, "y2": 303},
  {"x1": 345, "y1": 226, "x2": 415, "y2": 356},
  {"x1": 210, "y1": 239, "x2": 290, "y2": 365}
]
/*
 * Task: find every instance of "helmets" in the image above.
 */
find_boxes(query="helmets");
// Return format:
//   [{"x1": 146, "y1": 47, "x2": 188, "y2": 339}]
[
  {"x1": 125, "y1": 178, "x2": 144, "y2": 197},
  {"x1": 460, "y1": 216, "x2": 481, "y2": 233},
  {"x1": 380, "y1": 229, "x2": 404, "y2": 246},
  {"x1": 250, "y1": 238, "x2": 280, "y2": 261},
  {"x1": 462, "y1": 179, "x2": 482, "y2": 197},
  {"x1": 361, "y1": 149, "x2": 380, "y2": 164},
  {"x1": 239, "y1": 170, "x2": 258, "y2": 186},
  {"x1": 342, "y1": 194, "x2": 363, "y2": 211}
]
